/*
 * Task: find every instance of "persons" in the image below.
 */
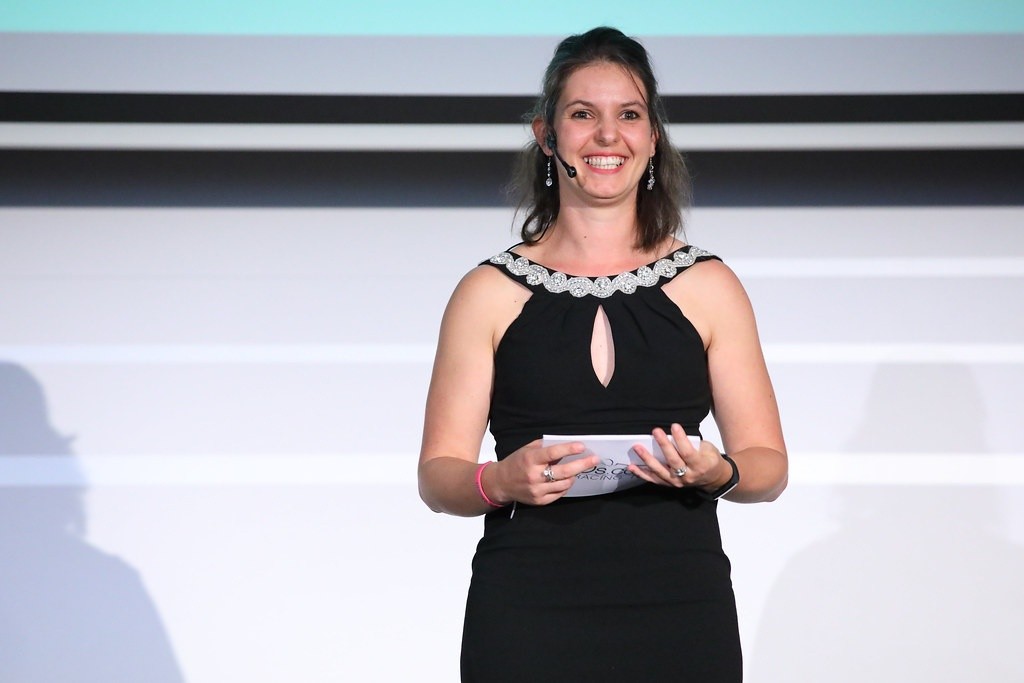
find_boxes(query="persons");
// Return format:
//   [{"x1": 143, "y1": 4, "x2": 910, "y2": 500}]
[{"x1": 418, "y1": 26, "x2": 788, "y2": 683}]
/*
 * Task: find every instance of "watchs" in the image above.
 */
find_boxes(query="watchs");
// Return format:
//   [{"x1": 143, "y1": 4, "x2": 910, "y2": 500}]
[{"x1": 697, "y1": 455, "x2": 740, "y2": 501}]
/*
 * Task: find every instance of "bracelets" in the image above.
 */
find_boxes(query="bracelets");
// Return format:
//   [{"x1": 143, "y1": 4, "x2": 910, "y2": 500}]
[{"x1": 476, "y1": 460, "x2": 510, "y2": 507}]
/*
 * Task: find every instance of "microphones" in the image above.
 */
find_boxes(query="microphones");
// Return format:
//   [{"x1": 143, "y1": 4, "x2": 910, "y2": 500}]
[{"x1": 547, "y1": 128, "x2": 578, "y2": 180}]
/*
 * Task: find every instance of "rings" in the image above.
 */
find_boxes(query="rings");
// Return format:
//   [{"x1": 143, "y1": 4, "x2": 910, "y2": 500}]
[
  {"x1": 671, "y1": 466, "x2": 687, "y2": 477},
  {"x1": 544, "y1": 465, "x2": 555, "y2": 482}
]
